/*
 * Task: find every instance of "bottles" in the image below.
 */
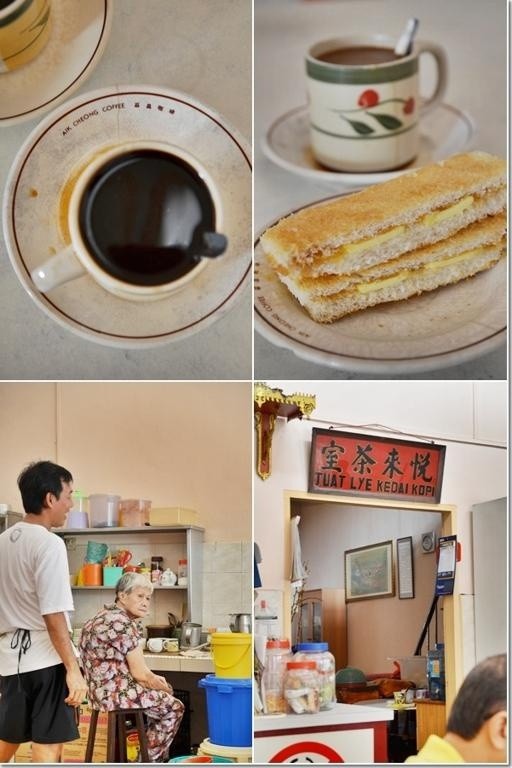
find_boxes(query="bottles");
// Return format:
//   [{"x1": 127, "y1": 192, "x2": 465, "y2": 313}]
[
  {"x1": 178, "y1": 559, "x2": 189, "y2": 586},
  {"x1": 260, "y1": 639, "x2": 295, "y2": 715},
  {"x1": 294, "y1": 641, "x2": 336, "y2": 711},
  {"x1": 283, "y1": 661, "x2": 320, "y2": 716},
  {"x1": 151, "y1": 556, "x2": 163, "y2": 587}
]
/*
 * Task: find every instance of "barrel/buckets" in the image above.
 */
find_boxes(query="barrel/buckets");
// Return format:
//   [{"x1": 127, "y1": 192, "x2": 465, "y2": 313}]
[
  {"x1": 168, "y1": 755, "x2": 234, "y2": 763},
  {"x1": 197, "y1": 738, "x2": 251, "y2": 762},
  {"x1": 426, "y1": 643, "x2": 447, "y2": 700},
  {"x1": 209, "y1": 632, "x2": 252, "y2": 680},
  {"x1": 197, "y1": 674, "x2": 251, "y2": 746}
]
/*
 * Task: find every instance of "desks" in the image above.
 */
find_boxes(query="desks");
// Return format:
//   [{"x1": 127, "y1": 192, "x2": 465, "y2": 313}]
[{"x1": 353, "y1": 699, "x2": 416, "y2": 735}]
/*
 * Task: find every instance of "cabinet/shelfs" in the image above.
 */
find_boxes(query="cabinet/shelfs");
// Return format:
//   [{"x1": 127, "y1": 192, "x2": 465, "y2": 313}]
[
  {"x1": 297, "y1": 588, "x2": 347, "y2": 674},
  {"x1": 49, "y1": 525, "x2": 204, "y2": 630}
]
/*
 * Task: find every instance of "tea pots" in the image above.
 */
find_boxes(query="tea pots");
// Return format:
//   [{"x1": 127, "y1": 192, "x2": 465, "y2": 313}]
[
  {"x1": 182, "y1": 623, "x2": 202, "y2": 648},
  {"x1": 159, "y1": 567, "x2": 177, "y2": 587}
]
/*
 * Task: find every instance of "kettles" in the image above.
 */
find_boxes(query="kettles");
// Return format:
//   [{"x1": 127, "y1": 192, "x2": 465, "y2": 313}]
[{"x1": 227, "y1": 613, "x2": 252, "y2": 633}]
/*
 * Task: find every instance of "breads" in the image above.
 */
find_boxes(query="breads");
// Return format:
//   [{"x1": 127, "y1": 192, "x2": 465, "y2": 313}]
[{"x1": 257, "y1": 151, "x2": 506, "y2": 325}]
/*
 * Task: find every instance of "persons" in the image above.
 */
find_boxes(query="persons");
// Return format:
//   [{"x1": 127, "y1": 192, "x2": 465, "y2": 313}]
[
  {"x1": 77, "y1": 571, "x2": 186, "y2": 761},
  {"x1": 0, "y1": 459, "x2": 86, "y2": 763},
  {"x1": 402, "y1": 653, "x2": 508, "y2": 764}
]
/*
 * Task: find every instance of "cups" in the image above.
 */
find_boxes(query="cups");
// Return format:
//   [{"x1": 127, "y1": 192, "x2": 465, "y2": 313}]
[
  {"x1": 163, "y1": 638, "x2": 179, "y2": 653},
  {"x1": 304, "y1": 33, "x2": 449, "y2": 174},
  {"x1": 68, "y1": 139, "x2": 224, "y2": 304},
  {"x1": 0, "y1": 0, "x2": 55, "y2": 79}
]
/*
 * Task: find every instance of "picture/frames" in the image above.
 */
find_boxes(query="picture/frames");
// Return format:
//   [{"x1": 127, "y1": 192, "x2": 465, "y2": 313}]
[
  {"x1": 397, "y1": 536, "x2": 414, "y2": 599},
  {"x1": 344, "y1": 540, "x2": 396, "y2": 603}
]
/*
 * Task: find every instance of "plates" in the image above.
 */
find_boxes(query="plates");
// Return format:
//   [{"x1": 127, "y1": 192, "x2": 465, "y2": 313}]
[
  {"x1": 0, "y1": 0, "x2": 114, "y2": 131},
  {"x1": 1, "y1": 83, "x2": 250, "y2": 353},
  {"x1": 259, "y1": 95, "x2": 478, "y2": 185},
  {"x1": 253, "y1": 186, "x2": 511, "y2": 378}
]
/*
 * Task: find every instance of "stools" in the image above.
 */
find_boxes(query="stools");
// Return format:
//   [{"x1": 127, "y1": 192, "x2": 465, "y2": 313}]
[{"x1": 84, "y1": 707, "x2": 149, "y2": 763}]
